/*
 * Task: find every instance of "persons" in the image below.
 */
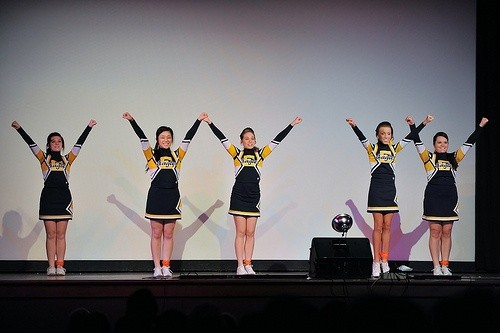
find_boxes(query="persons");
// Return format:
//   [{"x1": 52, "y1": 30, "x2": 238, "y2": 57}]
[
  {"x1": 202, "y1": 115, "x2": 302, "y2": 276},
  {"x1": 345, "y1": 113, "x2": 434, "y2": 278},
  {"x1": 10, "y1": 118, "x2": 98, "y2": 277},
  {"x1": 122, "y1": 111, "x2": 208, "y2": 277},
  {"x1": 404, "y1": 115, "x2": 492, "y2": 277}
]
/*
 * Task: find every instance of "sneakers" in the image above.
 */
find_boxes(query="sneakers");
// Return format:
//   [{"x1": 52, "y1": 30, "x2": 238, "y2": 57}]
[
  {"x1": 237, "y1": 266, "x2": 247, "y2": 275},
  {"x1": 161, "y1": 266, "x2": 173, "y2": 277},
  {"x1": 431, "y1": 264, "x2": 443, "y2": 275},
  {"x1": 47, "y1": 267, "x2": 57, "y2": 276},
  {"x1": 381, "y1": 260, "x2": 390, "y2": 274},
  {"x1": 372, "y1": 261, "x2": 381, "y2": 277},
  {"x1": 442, "y1": 266, "x2": 453, "y2": 276},
  {"x1": 244, "y1": 264, "x2": 256, "y2": 275},
  {"x1": 153, "y1": 267, "x2": 163, "y2": 277},
  {"x1": 56, "y1": 267, "x2": 66, "y2": 276}
]
